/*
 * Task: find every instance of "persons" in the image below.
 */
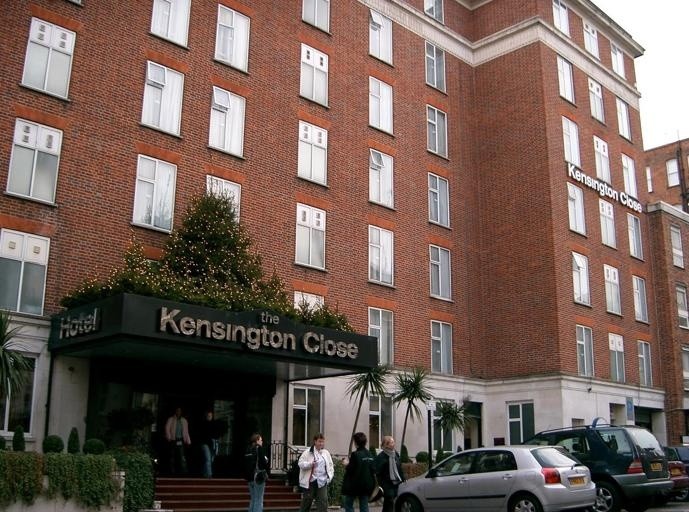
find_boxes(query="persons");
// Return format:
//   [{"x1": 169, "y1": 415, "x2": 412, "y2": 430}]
[
  {"x1": 198, "y1": 410, "x2": 219, "y2": 478},
  {"x1": 165, "y1": 405, "x2": 192, "y2": 476},
  {"x1": 243, "y1": 433, "x2": 270, "y2": 512},
  {"x1": 339, "y1": 431, "x2": 375, "y2": 512},
  {"x1": 297, "y1": 432, "x2": 335, "y2": 512},
  {"x1": 372, "y1": 434, "x2": 406, "y2": 511}
]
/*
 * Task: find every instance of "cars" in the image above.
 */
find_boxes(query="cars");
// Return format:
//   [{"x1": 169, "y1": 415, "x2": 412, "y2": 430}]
[{"x1": 391, "y1": 444, "x2": 689, "y2": 512}]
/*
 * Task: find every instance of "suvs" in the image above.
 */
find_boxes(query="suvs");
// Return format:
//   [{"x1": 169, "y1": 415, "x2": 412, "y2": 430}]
[{"x1": 512, "y1": 419, "x2": 675, "y2": 512}]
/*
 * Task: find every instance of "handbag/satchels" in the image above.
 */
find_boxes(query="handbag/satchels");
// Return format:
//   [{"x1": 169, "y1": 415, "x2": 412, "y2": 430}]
[
  {"x1": 367, "y1": 485, "x2": 384, "y2": 503},
  {"x1": 254, "y1": 468, "x2": 269, "y2": 485}
]
